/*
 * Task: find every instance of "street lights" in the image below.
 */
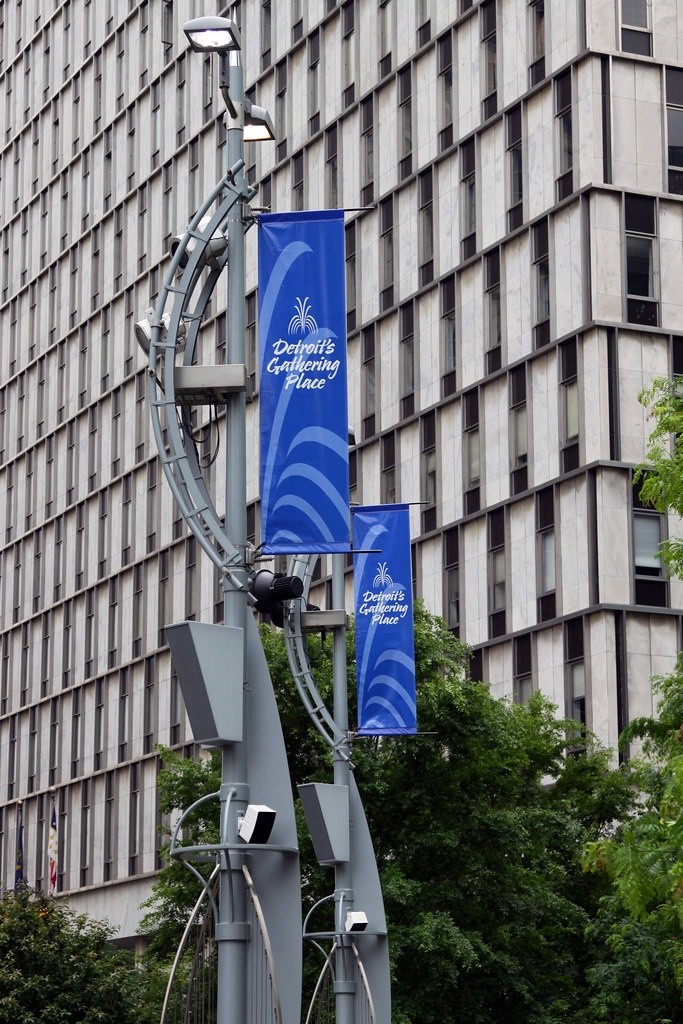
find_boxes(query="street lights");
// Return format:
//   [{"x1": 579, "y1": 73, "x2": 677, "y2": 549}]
[{"x1": 184, "y1": 10, "x2": 281, "y2": 1024}]
